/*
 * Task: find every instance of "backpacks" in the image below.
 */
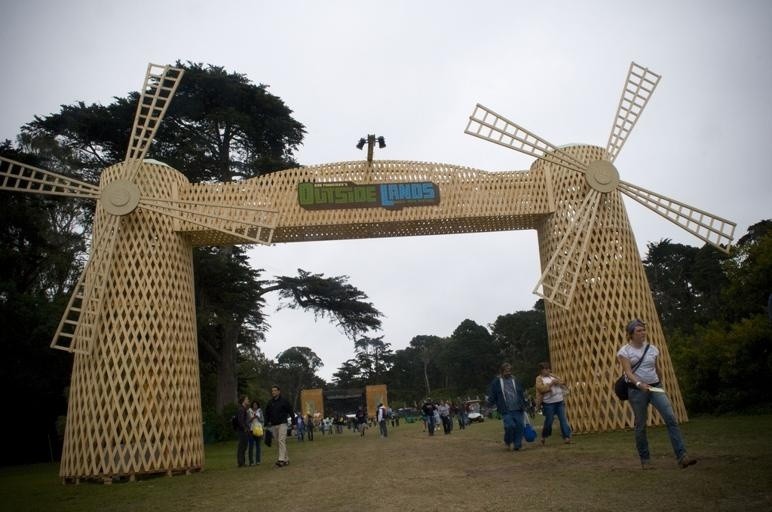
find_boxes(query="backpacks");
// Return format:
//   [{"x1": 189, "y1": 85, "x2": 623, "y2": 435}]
[{"x1": 232, "y1": 415, "x2": 238, "y2": 431}]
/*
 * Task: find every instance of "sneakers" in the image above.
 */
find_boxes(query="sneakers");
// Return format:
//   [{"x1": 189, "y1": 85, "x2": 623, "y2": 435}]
[
  {"x1": 679, "y1": 455, "x2": 697, "y2": 468},
  {"x1": 642, "y1": 462, "x2": 657, "y2": 469},
  {"x1": 276, "y1": 461, "x2": 284, "y2": 466}
]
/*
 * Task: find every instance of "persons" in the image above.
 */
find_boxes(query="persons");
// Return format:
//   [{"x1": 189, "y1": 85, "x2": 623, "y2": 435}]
[
  {"x1": 419, "y1": 397, "x2": 471, "y2": 437},
  {"x1": 535, "y1": 363, "x2": 572, "y2": 444},
  {"x1": 286, "y1": 401, "x2": 399, "y2": 441},
  {"x1": 616, "y1": 320, "x2": 697, "y2": 469},
  {"x1": 528, "y1": 394, "x2": 536, "y2": 419},
  {"x1": 264, "y1": 385, "x2": 296, "y2": 466},
  {"x1": 488, "y1": 362, "x2": 528, "y2": 450},
  {"x1": 235, "y1": 395, "x2": 251, "y2": 468},
  {"x1": 247, "y1": 400, "x2": 264, "y2": 466}
]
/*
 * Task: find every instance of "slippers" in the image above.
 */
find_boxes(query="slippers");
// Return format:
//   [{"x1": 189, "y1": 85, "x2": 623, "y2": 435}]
[
  {"x1": 564, "y1": 440, "x2": 575, "y2": 444},
  {"x1": 541, "y1": 438, "x2": 545, "y2": 445}
]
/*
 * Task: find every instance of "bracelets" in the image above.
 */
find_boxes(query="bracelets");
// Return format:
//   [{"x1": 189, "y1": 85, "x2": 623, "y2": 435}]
[{"x1": 636, "y1": 382, "x2": 641, "y2": 387}]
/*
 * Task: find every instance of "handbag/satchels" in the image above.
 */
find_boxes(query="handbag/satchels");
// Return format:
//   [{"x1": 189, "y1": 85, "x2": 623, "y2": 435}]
[
  {"x1": 615, "y1": 375, "x2": 628, "y2": 400},
  {"x1": 265, "y1": 424, "x2": 272, "y2": 446},
  {"x1": 523, "y1": 411, "x2": 536, "y2": 442},
  {"x1": 252, "y1": 422, "x2": 263, "y2": 436}
]
